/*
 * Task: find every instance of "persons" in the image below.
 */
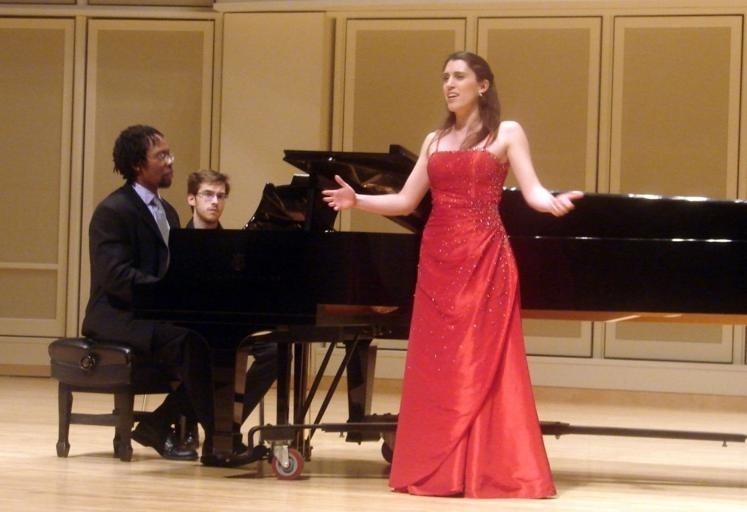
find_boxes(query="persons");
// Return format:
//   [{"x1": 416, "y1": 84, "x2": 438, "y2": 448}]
[
  {"x1": 176, "y1": 170, "x2": 289, "y2": 448},
  {"x1": 82, "y1": 126, "x2": 268, "y2": 467},
  {"x1": 323, "y1": 51, "x2": 584, "y2": 499}
]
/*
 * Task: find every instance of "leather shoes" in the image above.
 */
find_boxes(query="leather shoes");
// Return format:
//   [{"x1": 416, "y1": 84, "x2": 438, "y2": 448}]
[
  {"x1": 131, "y1": 413, "x2": 198, "y2": 461},
  {"x1": 201, "y1": 440, "x2": 268, "y2": 469},
  {"x1": 176, "y1": 417, "x2": 201, "y2": 451}
]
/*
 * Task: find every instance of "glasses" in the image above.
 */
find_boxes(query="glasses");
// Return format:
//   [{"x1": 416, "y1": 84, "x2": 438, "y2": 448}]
[
  {"x1": 195, "y1": 189, "x2": 230, "y2": 202},
  {"x1": 146, "y1": 149, "x2": 176, "y2": 164}
]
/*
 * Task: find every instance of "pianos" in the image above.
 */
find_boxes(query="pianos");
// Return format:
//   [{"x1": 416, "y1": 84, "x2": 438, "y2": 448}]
[{"x1": 132, "y1": 144, "x2": 747, "y2": 467}]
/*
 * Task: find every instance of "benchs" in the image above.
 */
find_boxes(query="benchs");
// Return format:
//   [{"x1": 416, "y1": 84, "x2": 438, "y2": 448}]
[{"x1": 49, "y1": 338, "x2": 278, "y2": 461}]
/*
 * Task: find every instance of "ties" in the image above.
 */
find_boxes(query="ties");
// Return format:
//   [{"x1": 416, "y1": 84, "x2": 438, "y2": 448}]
[{"x1": 152, "y1": 197, "x2": 170, "y2": 247}]
